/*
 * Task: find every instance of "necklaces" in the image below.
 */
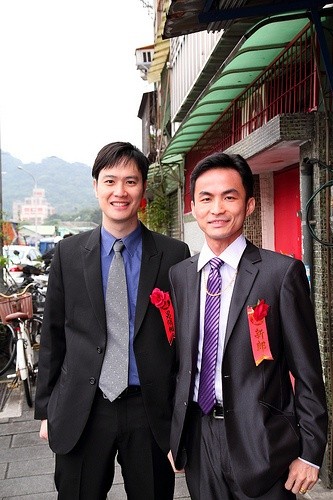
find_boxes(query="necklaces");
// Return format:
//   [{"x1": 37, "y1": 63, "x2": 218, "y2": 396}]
[{"x1": 204, "y1": 268, "x2": 237, "y2": 296}]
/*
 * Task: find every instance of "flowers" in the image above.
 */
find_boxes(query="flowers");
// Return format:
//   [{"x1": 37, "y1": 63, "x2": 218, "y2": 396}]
[
  {"x1": 150, "y1": 288, "x2": 165, "y2": 308},
  {"x1": 253, "y1": 299, "x2": 269, "y2": 321}
]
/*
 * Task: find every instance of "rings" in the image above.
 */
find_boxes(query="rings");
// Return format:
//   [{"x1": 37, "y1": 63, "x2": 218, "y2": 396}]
[{"x1": 300, "y1": 488, "x2": 307, "y2": 494}]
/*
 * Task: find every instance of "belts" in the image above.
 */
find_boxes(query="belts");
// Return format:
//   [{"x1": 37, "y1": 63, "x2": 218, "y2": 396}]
[
  {"x1": 192, "y1": 398, "x2": 227, "y2": 421},
  {"x1": 92, "y1": 384, "x2": 141, "y2": 408}
]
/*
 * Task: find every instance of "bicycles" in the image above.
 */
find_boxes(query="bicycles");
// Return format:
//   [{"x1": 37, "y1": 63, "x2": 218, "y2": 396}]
[{"x1": 0, "y1": 265, "x2": 49, "y2": 408}]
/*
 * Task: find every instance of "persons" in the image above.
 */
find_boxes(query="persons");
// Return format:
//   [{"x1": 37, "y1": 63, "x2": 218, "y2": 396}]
[
  {"x1": 33, "y1": 143, "x2": 191, "y2": 500},
  {"x1": 169, "y1": 153, "x2": 328, "y2": 500}
]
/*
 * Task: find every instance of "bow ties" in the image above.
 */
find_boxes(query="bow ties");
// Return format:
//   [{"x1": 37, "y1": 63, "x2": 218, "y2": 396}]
[
  {"x1": 196, "y1": 256, "x2": 225, "y2": 415},
  {"x1": 98, "y1": 240, "x2": 130, "y2": 402}
]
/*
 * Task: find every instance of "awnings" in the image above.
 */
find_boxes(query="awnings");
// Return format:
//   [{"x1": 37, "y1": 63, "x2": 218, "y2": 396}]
[
  {"x1": 161, "y1": 11, "x2": 325, "y2": 165},
  {"x1": 146, "y1": 160, "x2": 183, "y2": 189},
  {"x1": 163, "y1": 0, "x2": 333, "y2": 39}
]
